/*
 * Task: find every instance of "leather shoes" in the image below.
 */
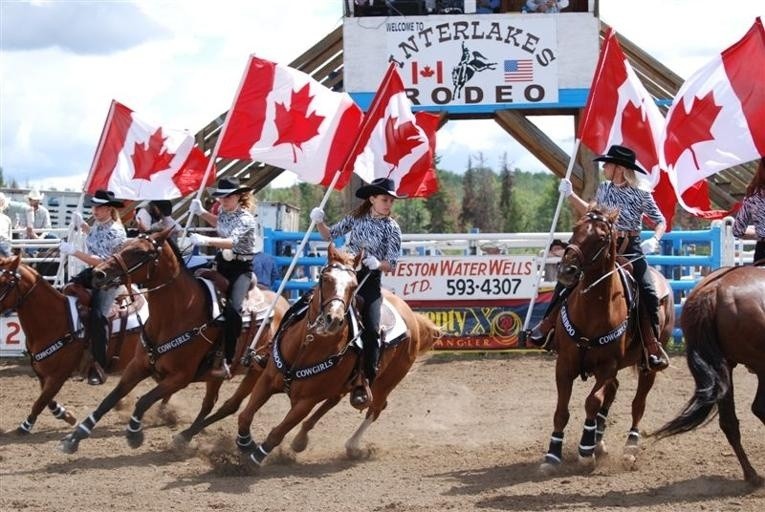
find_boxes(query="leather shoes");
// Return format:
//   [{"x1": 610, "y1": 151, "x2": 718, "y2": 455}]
[
  {"x1": 529, "y1": 335, "x2": 552, "y2": 351},
  {"x1": 641, "y1": 355, "x2": 668, "y2": 376}
]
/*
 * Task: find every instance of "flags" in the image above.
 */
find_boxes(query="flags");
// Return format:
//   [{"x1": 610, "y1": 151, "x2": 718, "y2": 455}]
[
  {"x1": 661, "y1": 17, "x2": 765, "y2": 221},
  {"x1": 216, "y1": 55, "x2": 366, "y2": 191},
  {"x1": 504, "y1": 59, "x2": 533, "y2": 82},
  {"x1": 82, "y1": 98, "x2": 217, "y2": 202},
  {"x1": 337, "y1": 59, "x2": 442, "y2": 200},
  {"x1": 576, "y1": 26, "x2": 677, "y2": 233},
  {"x1": 412, "y1": 60, "x2": 443, "y2": 85}
]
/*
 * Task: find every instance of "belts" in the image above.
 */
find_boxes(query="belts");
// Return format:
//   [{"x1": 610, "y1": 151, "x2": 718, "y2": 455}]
[
  {"x1": 220, "y1": 248, "x2": 253, "y2": 261},
  {"x1": 617, "y1": 231, "x2": 640, "y2": 238}
]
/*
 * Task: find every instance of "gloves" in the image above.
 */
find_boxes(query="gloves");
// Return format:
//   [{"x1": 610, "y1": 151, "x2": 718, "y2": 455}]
[
  {"x1": 71, "y1": 211, "x2": 82, "y2": 227},
  {"x1": 188, "y1": 232, "x2": 209, "y2": 246},
  {"x1": 59, "y1": 241, "x2": 76, "y2": 255},
  {"x1": 558, "y1": 177, "x2": 573, "y2": 197},
  {"x1": 363, "y1": 255, "x2": 381, "y2": 270},
  {"x1": 310, "y1": 206, "x2": 325, "y2": 224},
  {"x1": 189, "y1": 198, "x2": 207, "y2": 216},
  {"x1": 639, "y1": 237, "x2": 656, "y2": 253}
]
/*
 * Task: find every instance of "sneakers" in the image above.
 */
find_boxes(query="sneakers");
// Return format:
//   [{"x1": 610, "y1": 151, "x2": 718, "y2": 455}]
[
  {"x1": 212, "y1": 363, "x2": 232, "y2": 377},
  {"x1": 353, "y1": 388, "x2": 368, "y2": 405},
  {"x1": 89, "y1": 374, "x2": 101, "y2": 384},
  {"x1": 251, "y1": 355, "x2": 267, "y2": 369}
]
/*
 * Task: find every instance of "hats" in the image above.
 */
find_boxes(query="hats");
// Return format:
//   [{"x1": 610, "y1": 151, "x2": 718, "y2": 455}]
[
  {"x1": 83, "y1": 189, "x2": 125, "y2": 207},
  {"x1": 24, "y1": 188, "x2": 44, "y2": 202},
  {"x1": 211, "y1": 176, "x2": 252, "y2": 198},
  {"x1": 549, "y1": 239, "x2": 568, "y2": 251},
  {"x1": 355, "y1": 178, "x2": 410, "y2": 200},
  {"x1": 592, "y1": 146, "x2": 647, "y2": 176},
  {"x1": 149, "y1": 200, "x2": 172, "y2": 215},
  {"x1": 0, "y1": 192, "x2": 12, "y2": 211}
]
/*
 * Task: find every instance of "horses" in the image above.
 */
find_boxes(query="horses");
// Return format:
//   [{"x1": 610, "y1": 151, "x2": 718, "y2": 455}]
[
  {"x1": 235, "y1": 240, "x2": 442, "y2": 469},
  {"x1": 645, "y1": 264, "x2": 765, "y2": 488},
  {"x1": 0, "y1": 250, "x2": 175, "y2": 436},
  {"x1": 61, "y1": 216, "x2": 292, "y2": 455},
  {"x1": 537, "y1": 197, "x2": 675, "y2": 476}
]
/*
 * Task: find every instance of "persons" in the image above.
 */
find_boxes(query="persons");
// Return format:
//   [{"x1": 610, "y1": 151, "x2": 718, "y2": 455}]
[
  {"x1": 476, "y1": 0, "x2": 501, "y2": 13},
  {"x1": 138, "y1": 200, "x2": 182, "y2": 233},
  {"x1": 0, "y1": 188, "x2": 58, "y2": 258},
  {"x1": 732, "y1": 157, "x2": 765, "y2": 266},
  {"x1": 526, "y1": 0, "x2": 570, "y2": 13},
  {"x1": 528, "y1": 145, "x2": 667, "y2": 371},
  {"x1": 189, "y1": 175, "x2": 257, "y2": 379},
  {"x1": 539, "y1": 239, "x2": 569, "y2": 281},
  {"x1": 59, "y1": 189, "x2": 126, "y2": 386},
  {"x1": 310, "y1": 178, "x2": 410, "y2": 405}
]
